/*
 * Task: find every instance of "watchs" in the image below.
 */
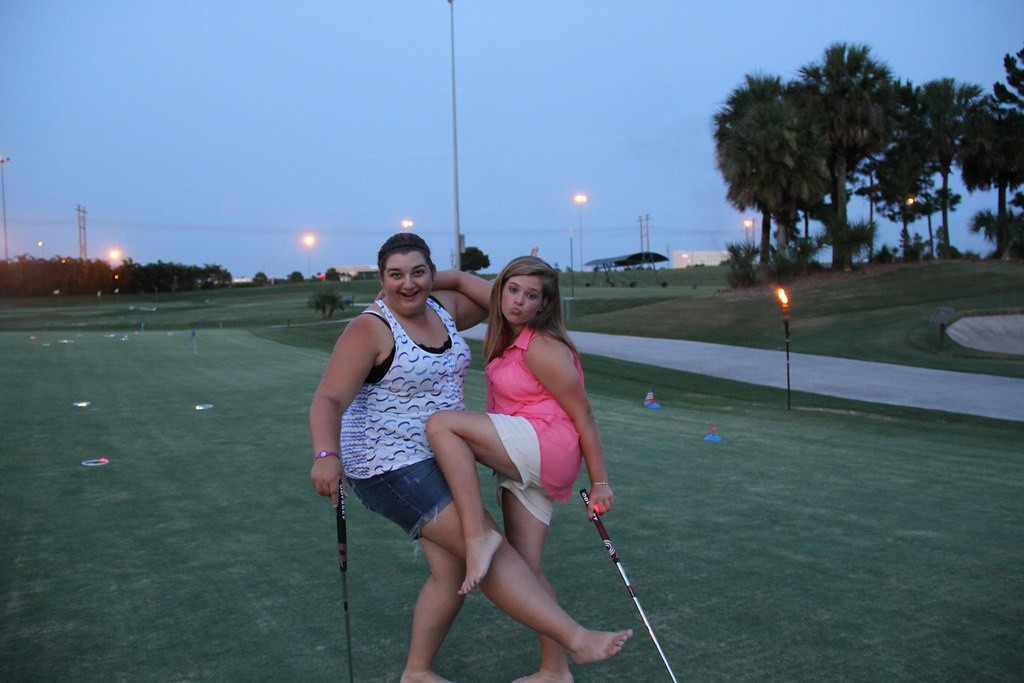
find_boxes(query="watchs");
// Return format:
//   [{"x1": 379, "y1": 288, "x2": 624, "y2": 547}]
[{"x1": 312, "y1": 451, "x2": 340, "y2": 460}]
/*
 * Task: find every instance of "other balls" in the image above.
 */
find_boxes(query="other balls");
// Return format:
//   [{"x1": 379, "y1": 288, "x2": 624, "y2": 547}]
[{"x1": 593, "y1": 502, "x2": 607, "y2": 515}]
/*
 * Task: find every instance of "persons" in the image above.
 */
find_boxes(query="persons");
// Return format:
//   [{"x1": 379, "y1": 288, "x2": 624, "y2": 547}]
[
  {"x1": 311, "y1": 233, "x2": 633, "y2": 683},
  {"x1": 379, "y1": 255, "x2": 615, "y2": 683}
]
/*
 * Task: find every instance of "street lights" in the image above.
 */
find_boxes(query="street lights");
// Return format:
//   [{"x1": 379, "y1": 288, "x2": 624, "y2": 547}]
[
  {"x1": 743, "y1": 218, "x2": 753, "y2": 244},
  {"x1": 574, "y1": 193, "x2": 587, "y2": 286},
  {"x1": 1, "y1": 157, "x2": 11, "y2": 262}
]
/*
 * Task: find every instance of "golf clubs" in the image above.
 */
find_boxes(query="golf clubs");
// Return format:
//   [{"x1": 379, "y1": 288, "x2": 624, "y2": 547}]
[
  {"x1": 337, "y1": 478, "x2": 354, "y2": 683},
  {"x1": 579, "y1": 487, "x2": 677, "y2": 683}
]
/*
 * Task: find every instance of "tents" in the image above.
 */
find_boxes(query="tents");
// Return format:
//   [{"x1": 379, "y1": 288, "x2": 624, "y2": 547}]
[{"x1": 584, "y1": 252, "x2": 668, "y2": 290}]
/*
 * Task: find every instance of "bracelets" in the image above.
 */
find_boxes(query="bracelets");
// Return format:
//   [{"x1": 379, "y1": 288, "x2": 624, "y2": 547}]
[{"x1": 591, "y1": 482, "x2": 609, "y2": 485}]
